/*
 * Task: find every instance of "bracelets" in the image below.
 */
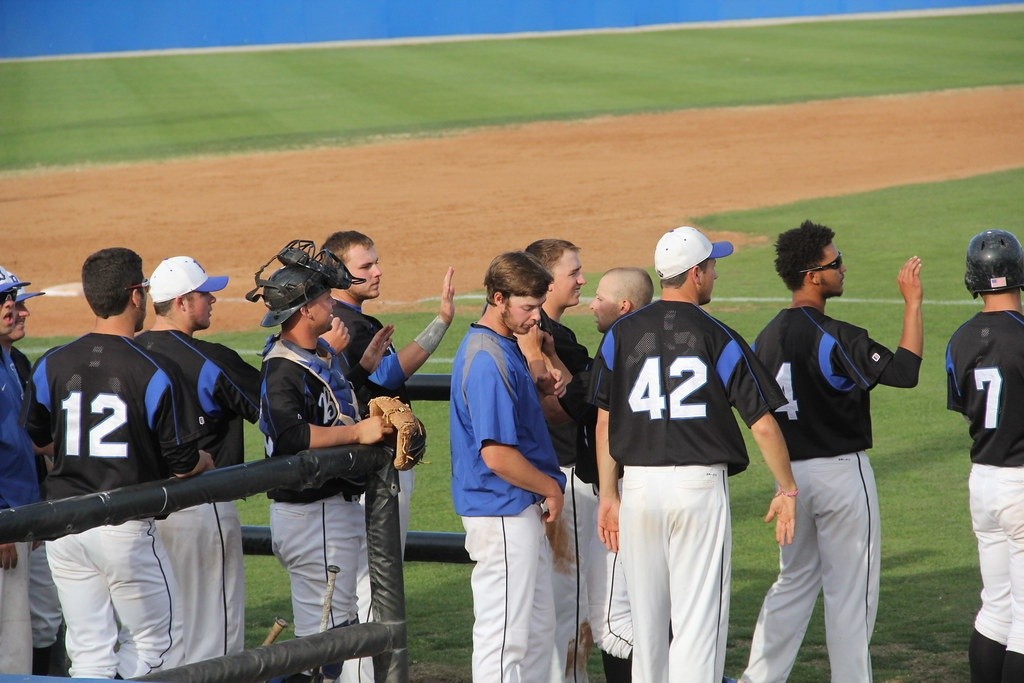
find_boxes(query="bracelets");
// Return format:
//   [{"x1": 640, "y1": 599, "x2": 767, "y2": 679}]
[{"x1": 775, "y1": 487, "x2": 799, "y2": 497}]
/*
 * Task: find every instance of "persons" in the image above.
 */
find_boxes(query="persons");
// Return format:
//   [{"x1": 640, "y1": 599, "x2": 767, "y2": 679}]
[
  {"x1": 451, "y1": 238, "x2": 672, "y2": 683},
  {"x1": 946, "y1": 228, "x2": 1024, "y2": 683},
  {"x1": 18, "y1": 249, "x2": 216, "y2": 678},
  {"x1": 248, "y1": 230, "x2": 456, "y2": 683},
  {"x1": 735, "y1": 220, "x2": 924, "y2": 683},
  {"x1": 0, "y1": 263, "x2": 64, "y2": 676},
  {"x1": 137, "y1": 255, "x2": 351, "y2": 666},
  {"x1": 577, "y1": 226, "x2": 800, "y2": 683}
]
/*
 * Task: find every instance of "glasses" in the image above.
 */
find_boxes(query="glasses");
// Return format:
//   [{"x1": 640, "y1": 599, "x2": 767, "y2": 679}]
[
  {"x1": 124, "y1": 279, "x2": 151, "y2": 293},
  {"x1": 0, "y1": 289, "x2": 17, "y2": 304},
  {"x1": 799, "y1": 251, "x2": 842, "y2": 272}
]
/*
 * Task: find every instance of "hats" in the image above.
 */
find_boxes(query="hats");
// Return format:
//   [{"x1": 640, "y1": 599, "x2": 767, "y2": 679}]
[
  {"x1": 260, "y1": 265, "x2": 331, "y2": 327},
  {"x1": 6, "y1": 271, "x2": 46, "y2": 302},
  {"x1": 149, "y1": 256, "x2": 229, "y2": 303},
  {"x1": 654, "y1": 226, "x2": 733, "y2": 281},
  {"x1": 0, "y1": 266, "x2": 31, "y2": 293}
]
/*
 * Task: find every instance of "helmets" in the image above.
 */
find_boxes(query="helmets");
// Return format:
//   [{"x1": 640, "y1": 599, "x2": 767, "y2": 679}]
[{"x1": 964, "y1": 230, "x2": 1024, "y2": 299}]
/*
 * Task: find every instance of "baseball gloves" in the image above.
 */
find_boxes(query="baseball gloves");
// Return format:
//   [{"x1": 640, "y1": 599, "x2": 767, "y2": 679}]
[{"x1": 368, "y1": 395, "x2": 428, "y2": 473}]
